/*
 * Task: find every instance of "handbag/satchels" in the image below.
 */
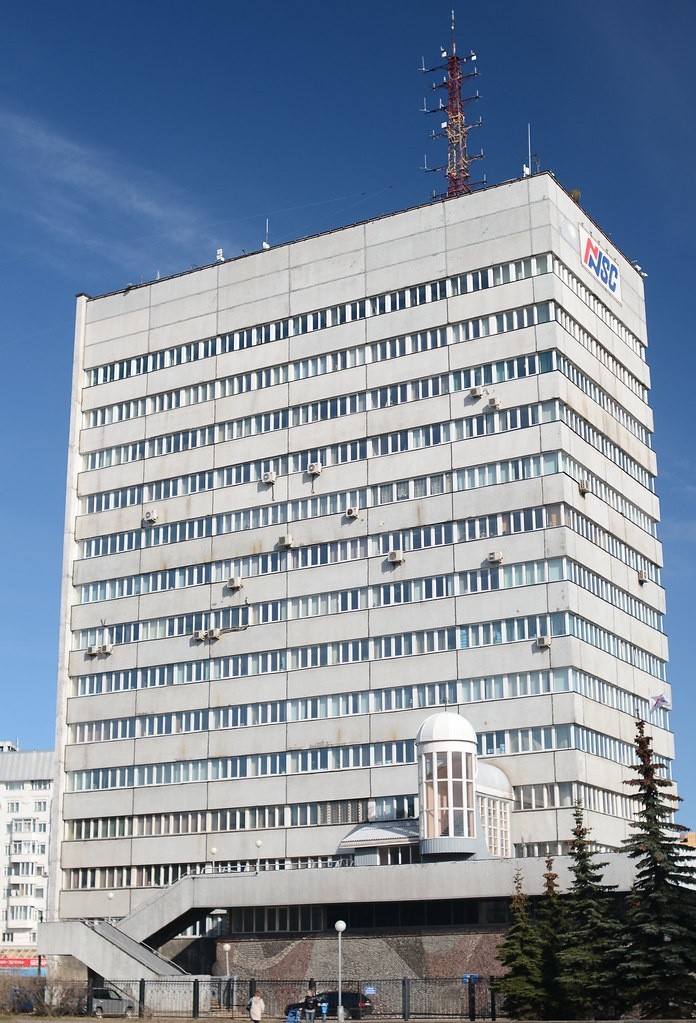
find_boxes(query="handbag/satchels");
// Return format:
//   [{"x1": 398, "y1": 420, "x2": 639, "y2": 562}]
[{"x1": 245, "y1": 998, "x2": 252, "y2": 1012}]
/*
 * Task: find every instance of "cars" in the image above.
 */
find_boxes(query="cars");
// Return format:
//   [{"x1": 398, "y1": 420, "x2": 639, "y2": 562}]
[
  {"x1": 284, "y1": 990, "x2": 374, "y2": 1020},
  {"x1": 61, "y1": 986, "x2": 134, "y2": 1017},
  {"x1": 11, "y1": 1001, "x2": 33, "y2": 1013}
]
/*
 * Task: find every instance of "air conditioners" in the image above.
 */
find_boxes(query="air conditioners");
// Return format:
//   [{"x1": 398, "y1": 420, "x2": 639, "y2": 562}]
[
  {"x1": 307, "y1": 462, "x2": 322, "y2": 475},
  {"x1": 487, "y1": 396, "x2": 501, "y2": 407},
  {"x1": 193, "y1": 630, "x2": 205, "y2": 640},
  {"x1": 579, "y1": 479, "x2": 591, "y2": 493},
  {"x1": 207, "y1": 628, "x2": 220, "y2": 638},
  {"x1": 87, "y1": 645, "x2": 99, "y2": 654},
  {"x1": 469, "y1": 387, "x2": 483, "y2": 398},
  {"x1": 345, "y1": 507, "x2": 358, "y2": 518},
  {"x1": 487, "y1": 550, "x2": 502, "y2": 562},
  {"x1": 227, "y1": 577, "x2": 241, "y2": 588},
  {"x1": 144, "y1": 510, "x2": 158, "y2": 522},
  {"x1": 388, "y1": 550, "x2": 403, "y2": 563},
  {"x1": 101, "y1": 644, "x2": 112, "y2": 653},
  {"x1": 278, "y1": 535, "x2": 293, "y2": 547},
  {"x1": 537, "y1": 636, "x2": 552, "y2": 648},
  {"x1": 638, "y1": 570, "x2": 649, "y2": 583},
  {"x1": 261, "y1": 471, "x2": 276, "y2": 484}
]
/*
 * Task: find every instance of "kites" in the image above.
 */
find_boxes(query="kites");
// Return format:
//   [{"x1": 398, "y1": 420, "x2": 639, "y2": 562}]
[{"x1": 649, "y1": 693, "x2": 670, "y2": 714}]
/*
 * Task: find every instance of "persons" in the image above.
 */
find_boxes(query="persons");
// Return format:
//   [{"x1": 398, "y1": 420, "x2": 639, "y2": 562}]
[
  {"x1": 248, "y1": 991, "x2": 265, "y2": 1023},
  {"x1": 299, "y1": 989, "x2": 321, "y2": 1023}
]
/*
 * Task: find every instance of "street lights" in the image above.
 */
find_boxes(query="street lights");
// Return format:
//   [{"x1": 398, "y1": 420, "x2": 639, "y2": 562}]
[
  {"x1": 222, "y1": 943, "x2": 231, "y2": 976},
  {"x1": 107, "y1": 892, "x2": 114, "y2": 922},
  {"x1": 335, "y1": 921, "x2": 346, "y2": 1023},
  {"x1": 30, "y1": 905, "x2": 43, "y2": 977},
  {"x1": 210, "y1": 847, "x2": 217, "y2": 874},
  {"x1": 255, "y1": 840, "x2": 263, "y2": 869}
]
